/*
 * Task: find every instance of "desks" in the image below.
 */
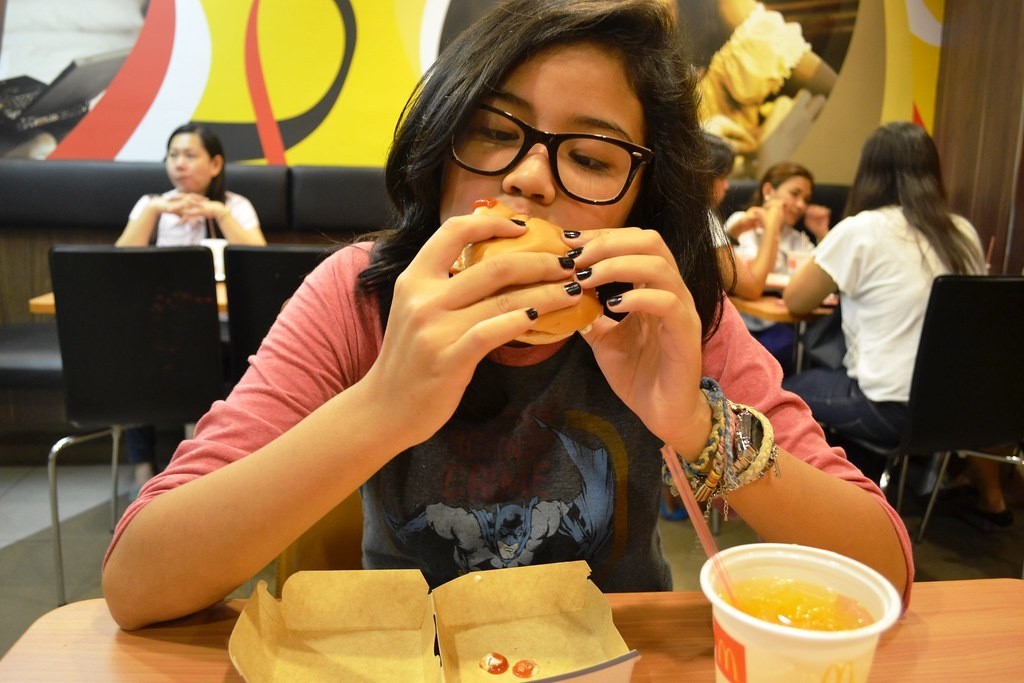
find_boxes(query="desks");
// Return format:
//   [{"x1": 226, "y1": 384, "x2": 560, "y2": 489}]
[{"x1": 729, "y1": 294, "x2": 837, "y2": 375}]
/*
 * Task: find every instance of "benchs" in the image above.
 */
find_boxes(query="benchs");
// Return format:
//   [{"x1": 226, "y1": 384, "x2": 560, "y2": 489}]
[
  {"x1": 1, "y1": 157, "x2": 396, "y2": 386},
  {"x1": 726, "y1": 176, "x2": 849, "y2": 245}
]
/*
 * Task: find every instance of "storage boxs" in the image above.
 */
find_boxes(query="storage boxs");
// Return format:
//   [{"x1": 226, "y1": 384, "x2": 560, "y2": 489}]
[{"x1": 228, "y1": 559, "x2": 642, "y2": 683}]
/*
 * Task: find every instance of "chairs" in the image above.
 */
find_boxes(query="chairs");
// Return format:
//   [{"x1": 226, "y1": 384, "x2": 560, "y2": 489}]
[
  {"x1": 854, "y1": 274, "x2": 1023, "y2": 542},
  {"x1": 47, "y1": 243, "x2": 230, "y2": 606},
  {"x1": 223, "y1": 241, "x2": 341, "y2": 388}
]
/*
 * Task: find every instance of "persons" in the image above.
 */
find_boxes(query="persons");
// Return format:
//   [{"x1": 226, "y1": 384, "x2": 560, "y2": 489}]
[
  {"x1": 783, "y1": 122, "x2": 1014, "y2": 527},
  {"x1": 103, "y1": 0, "x2": 914, "y2": 631},
  {"x1": 725, "y1": 162, "x2": 832, "y2": 275},
  {"x1": 697, "y1": 131, "x2": 795, "y2": 378},
  {"x1": 115, "y1": 125, "x2": 266, "y2": 501}
]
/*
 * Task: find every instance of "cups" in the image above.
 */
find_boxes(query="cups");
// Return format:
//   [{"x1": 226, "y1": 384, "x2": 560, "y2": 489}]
[
  {"x1": 699, "y1": 544, "x2": 903, "y2": 682},
  {"x1": 787, "y1": 251, "x2": 808, "y2": 276},
  {"x1": 200, "y1": 237, "x2": 228, "y2": 283}
]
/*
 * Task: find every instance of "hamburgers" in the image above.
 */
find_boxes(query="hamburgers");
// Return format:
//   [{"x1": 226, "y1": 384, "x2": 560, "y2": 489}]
[{"x1": 449, "y1": 198, "x2": 605, "y2": 345}]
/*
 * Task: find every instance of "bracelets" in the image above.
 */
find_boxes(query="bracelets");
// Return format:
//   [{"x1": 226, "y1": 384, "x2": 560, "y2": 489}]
[
  {"x1": 662, "y1": 378, "x2": 780, "y2": 548},
  {"x1": 727, "y1": 232, "x2": 740, "y2": 247}
]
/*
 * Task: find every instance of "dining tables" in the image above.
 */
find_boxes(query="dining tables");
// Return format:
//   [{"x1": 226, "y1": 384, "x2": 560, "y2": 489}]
[{"x1": 0, "y1": 579, "x2": 1024, "y2": 683}]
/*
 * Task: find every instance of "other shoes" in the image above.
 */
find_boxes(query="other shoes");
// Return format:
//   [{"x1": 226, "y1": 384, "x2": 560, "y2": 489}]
[
  {"x1": 128, "y1": 479, "x2": 144, "y2": 501},
  {"x1": 920, "y1": 481, "x2": 980, "y2": 506},
  {"x1": 970, "y1": 504, "x2": 1015, "y2": 527}
]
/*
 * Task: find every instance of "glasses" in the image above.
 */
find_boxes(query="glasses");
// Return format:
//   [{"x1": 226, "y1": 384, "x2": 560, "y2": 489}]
[{"x1": 449, "y1": 108, "x2": 654, "y2": 205}]
